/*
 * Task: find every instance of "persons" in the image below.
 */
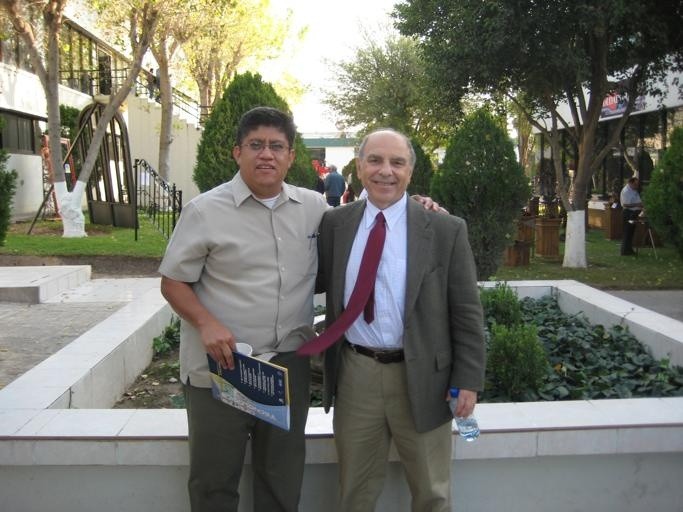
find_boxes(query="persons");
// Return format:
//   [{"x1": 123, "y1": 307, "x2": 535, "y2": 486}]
[
  {"x1": 148, "y1": 68, "x2": 161, "y2": 103},
  {"x1": 69, "y1": 71, "x2": 91, "y2": 94},
  {"x1": 313, "y1": 127, "x2": 488, "y2": 512},
  {"x1": 157, "y1": 106, "x2": 450, "y2": 512},
  {"x1": 312, "y1": 164, "x2": 355, "y2": 207},
  {"x1": 619, "y1": 177, "x2": 642, "y2": 256}
]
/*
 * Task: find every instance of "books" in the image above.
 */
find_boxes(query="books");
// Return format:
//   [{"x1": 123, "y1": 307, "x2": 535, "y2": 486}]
[{"x1": 206, "y1": 350, "x2": 291, "y2": 431}]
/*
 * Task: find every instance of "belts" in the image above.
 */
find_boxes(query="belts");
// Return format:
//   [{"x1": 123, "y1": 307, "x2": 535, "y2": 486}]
[{"x1": 349, "y1": 343, "x2": 404, "y2": 363}]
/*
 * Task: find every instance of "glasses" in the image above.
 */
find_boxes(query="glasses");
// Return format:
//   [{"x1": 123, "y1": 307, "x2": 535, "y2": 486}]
[{"x1": 240, "y1": 141, "x2": 290, "y2": 153}]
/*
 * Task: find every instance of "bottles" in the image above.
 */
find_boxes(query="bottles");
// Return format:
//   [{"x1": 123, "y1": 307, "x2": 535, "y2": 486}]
[{"x1": 449, "y1": 387, "x2": 481, "y2": 442}]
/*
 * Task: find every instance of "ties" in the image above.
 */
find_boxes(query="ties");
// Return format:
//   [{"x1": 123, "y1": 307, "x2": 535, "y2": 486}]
[{"x1": 296, "y1": 212, "x2": 385, "y2": 356}]
